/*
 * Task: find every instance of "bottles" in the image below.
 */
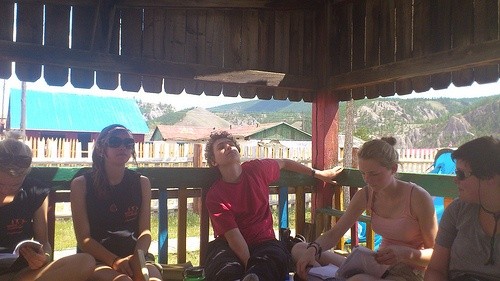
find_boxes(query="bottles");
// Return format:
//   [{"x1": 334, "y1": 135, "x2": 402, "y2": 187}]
[{"x1": 183, "y1": 267, "x2": 206, "y2": 281}]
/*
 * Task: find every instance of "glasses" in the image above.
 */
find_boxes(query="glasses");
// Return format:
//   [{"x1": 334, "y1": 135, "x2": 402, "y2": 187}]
[
  {"x1": 0, "y1": 153, "x2": 32, "y2": 168},
  {"x1": 108, "y1": 137, "x2": 136, "y2": 149},
  {"x1": 455, "y1": 169, "x2": 480, "y2": 180}
]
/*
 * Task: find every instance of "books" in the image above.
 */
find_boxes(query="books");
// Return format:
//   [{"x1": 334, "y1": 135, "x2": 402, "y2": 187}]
[{"x1": 0, "y1": 239, "x2": 44, "y2": 271}]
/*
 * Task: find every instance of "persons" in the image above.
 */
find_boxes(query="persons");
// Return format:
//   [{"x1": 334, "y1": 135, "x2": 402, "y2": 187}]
[
  {"x1": 70, "y1": 124, "x2": 164, "y2": 281},
  {"x1": 291, "y1": 137, "x2": 440, "y2": 281},
  {"x1": 200, "y1": 131, "x2": 345, "y2": 281},
  {"x1": 424, "y1": 136, "x2": 500, "y2": 281},
  {"x1": 0, "y1": 140, "x2": 96, "y2": 281}
]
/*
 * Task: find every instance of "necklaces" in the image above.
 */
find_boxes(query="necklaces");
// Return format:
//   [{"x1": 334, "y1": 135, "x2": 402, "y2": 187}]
[
  {"x1": 481, "y1": 206, "x2": 500, "y2": 220},
  {"x1": 307, "y1": 240, "x2": 323, "y2": 257}
]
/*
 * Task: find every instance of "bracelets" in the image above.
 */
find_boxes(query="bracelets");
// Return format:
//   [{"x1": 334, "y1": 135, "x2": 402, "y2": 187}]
[
  {"x1": 110, "y1": 257, "x2": 119, "y2": 267},
  {"x1": 311, "y1": 169, "x2": 316, "y2": 179}
]
